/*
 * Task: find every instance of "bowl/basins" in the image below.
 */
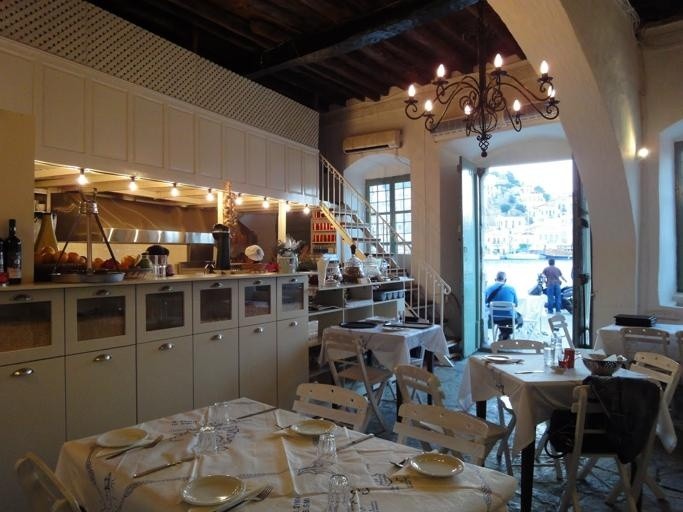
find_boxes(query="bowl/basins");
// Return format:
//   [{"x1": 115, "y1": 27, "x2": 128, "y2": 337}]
[
  {"x1": 581, "y1": 353, "x2": 628, "y2": 377},
  {"x1": 242, "y1": 263, "x2": 267, "y2": 271},
  {"x1": 374, "y1": 288, "x2": 405, "y2": 301}
]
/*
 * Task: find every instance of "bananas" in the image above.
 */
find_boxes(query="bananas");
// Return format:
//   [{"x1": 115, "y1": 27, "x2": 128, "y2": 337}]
[{"x1": 120, "y1": 253, "x2": 142, "y2": 270}]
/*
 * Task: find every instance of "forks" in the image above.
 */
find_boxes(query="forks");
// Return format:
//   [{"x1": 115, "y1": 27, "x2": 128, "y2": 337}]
[
  {"x1": 214, "y1": 484, "x2": 274, "y2": 511},
  {"x1": 104, "y1": 434, "x2": 164, "y2": 462},
  {"x1": 387, "y1": 448, "x2": 438, "y2": 468}
]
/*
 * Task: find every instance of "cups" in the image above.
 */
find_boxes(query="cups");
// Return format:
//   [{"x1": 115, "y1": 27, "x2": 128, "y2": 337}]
[
  {"x1": 194, "y1": 426, "x2": 220, "y2": 454},
  {"x1": 543, "y1": 341, "x2": 570, "y2": 370},
  {"x1": 208, "y1": 400, "x2": 229, "y2": 425},
  {"x1": 155, "y1": 253, "x2": 168, "y2": 280},
  {"x1": 278, "y1": 257, "x2": 297, "y2": 275},
  {"x1": 397, "y1": 309, "x2": 405, "y2": 325},
  {"x1": 390, "y1": 319, "x2": 401, "y2": 326},
  {"x1": 315, "y1": 431, "x2": 339, "y2": 466},
  {"x1": 326, "y1": 474, "x2": 352, "y2": 512}
]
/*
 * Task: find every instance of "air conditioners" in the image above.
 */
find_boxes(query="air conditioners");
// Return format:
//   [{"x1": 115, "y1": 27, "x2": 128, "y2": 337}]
[{"x1": 342, "y1": 130, "x2": 401, "y2": 155}]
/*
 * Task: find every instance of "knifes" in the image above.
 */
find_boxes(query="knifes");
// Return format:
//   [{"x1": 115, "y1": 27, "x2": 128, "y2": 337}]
[
  {"x1": 236, "y1": 406, "x2": 280, "y2": 419},
  {"x1": 337, "y1": 433, "x2": 374, "y2": 452},
  {"x1": 514, "y1": 370, "x2": 545, "y2": 375},
  {"x1": 132, "y1": 454, "x2": 198, "y2": 478}
]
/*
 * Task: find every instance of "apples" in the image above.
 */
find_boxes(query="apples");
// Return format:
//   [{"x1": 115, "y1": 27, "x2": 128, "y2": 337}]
[{"x1": 42, "y1": 245, "x2": 103, "y2": 268}]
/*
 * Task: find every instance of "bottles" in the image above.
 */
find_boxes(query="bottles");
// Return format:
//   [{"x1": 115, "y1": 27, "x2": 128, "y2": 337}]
[
  {"x1": 35, "y1": 212, "x2": 59, "y2": 281},
  {"x1": 549, "y1": 327, "x2": 564, "y2": 365},
  {"x1": 139, "y1": 252, "x2": 153, "y2": 270},
  {"x1": 1, "y1": 218, "x2": 25, "y2": 285},
  {"x1": 346, "y1": 244, "x2": 379, "y2": 282}
]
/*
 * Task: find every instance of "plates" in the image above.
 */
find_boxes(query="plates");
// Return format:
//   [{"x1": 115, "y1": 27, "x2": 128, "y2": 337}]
[
  {"x1": 289, "y1": 418, "x2": 337, "y2": 437},
  {"x1": 410, "y1": 452, "x2": 465, "y2": 480},
  {"x1": 366, "y1": 317, "x2": 389, "y2": 324},
  {"x1": 381, "y1": 326, "x2": 405, "y2": 332},
  {"x1": 484, "y1": 353, "x2": 512, "y2": 362},
  {"x1": 96, "y1": 427, "x2": 149, "y2": 448},
  {"x1": 182, "y1": 473, "x2": 245, "y2": 507}
]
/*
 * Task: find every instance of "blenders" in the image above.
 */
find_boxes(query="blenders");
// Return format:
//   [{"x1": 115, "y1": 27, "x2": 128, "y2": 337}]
[{"x1": 317, "y1": 253, "x2": 343, "y2": 290}]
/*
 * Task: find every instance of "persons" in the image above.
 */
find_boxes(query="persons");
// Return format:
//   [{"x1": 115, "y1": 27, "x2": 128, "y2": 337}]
[
  {"x1": 541, "y1": 258, "x2": 566, "y2": 314},
  {"x1": 485, "y1": 271, "x2": 523, "y2": 341}
]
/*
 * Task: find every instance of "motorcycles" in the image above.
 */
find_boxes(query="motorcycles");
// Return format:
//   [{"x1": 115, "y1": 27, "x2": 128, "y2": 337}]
[{"x1": 538, "y1": 281, "x2": 572, "y2": 316}]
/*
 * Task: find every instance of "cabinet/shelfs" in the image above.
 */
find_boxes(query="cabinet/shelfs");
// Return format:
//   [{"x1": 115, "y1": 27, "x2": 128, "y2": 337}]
[
  {"x1": 308, "y1": 274, "x2": 415, "y2": 409},
  {"x1": 310, "y1": 215, "x2": 341, "y2": 271}
]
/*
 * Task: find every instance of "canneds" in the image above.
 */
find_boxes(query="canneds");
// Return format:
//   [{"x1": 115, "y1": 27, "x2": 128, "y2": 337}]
[{"x1": 565, "y1": 348, "x2": 575, "y2": 369}]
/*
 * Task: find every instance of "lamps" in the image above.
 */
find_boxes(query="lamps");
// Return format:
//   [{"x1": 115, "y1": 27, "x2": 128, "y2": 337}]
[{"x1": 403, "y1": 0, "x2": 560, "y2": 158}]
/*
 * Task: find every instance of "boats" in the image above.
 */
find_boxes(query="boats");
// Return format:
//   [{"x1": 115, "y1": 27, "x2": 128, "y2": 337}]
[
  {"x1": 538, "y1": 243, "x2": 572, "y2": 260},
  {"x1": 498, "y1": 252, "x2": 537, "y2": 260}
]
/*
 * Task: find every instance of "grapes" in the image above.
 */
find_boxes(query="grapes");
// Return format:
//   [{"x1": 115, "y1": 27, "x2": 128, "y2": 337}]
[{"x1": 104, "y1": 257, "x2": 119, "y2": 270}]
